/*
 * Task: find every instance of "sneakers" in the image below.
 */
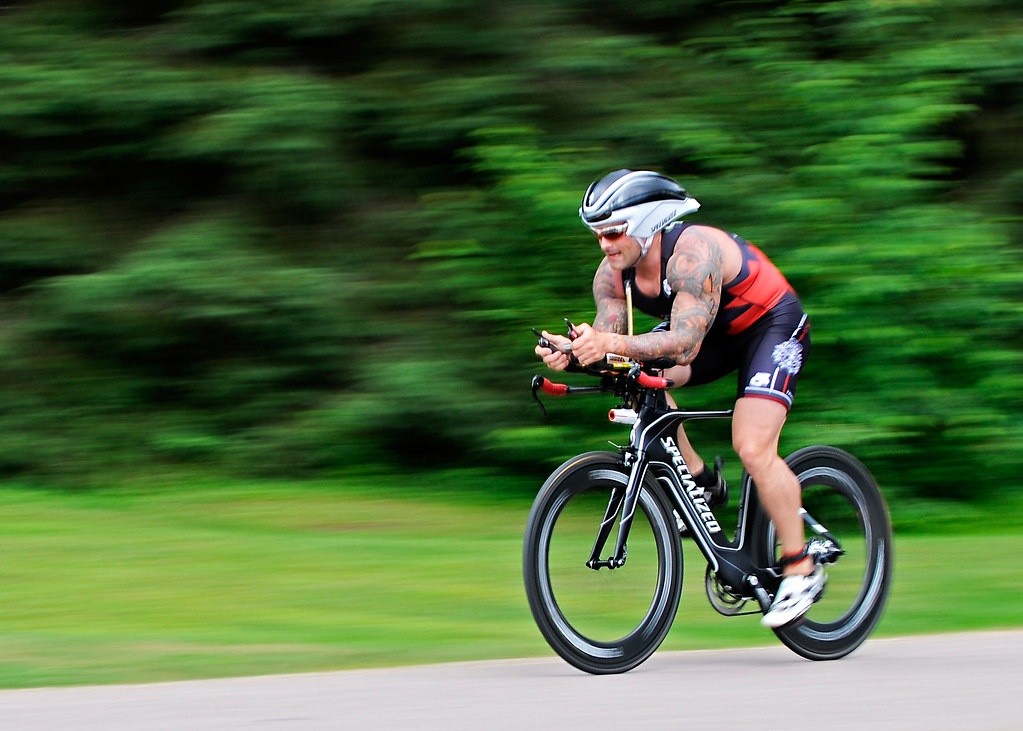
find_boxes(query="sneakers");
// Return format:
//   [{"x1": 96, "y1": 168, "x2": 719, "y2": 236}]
[
  {"x1": 671, "y1": 466, "x2": 729, "y2": 533},
  {"x1": 760, "y1": 558, "x2": 830, "y2": 628}
]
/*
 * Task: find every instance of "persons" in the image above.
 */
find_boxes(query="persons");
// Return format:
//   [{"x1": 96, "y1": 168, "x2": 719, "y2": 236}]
[{"x1": 534, "y1": 165, "x2": 830, "y2": 627}]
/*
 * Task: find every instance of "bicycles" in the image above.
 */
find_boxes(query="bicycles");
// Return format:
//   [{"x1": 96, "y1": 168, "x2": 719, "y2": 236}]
[{"x1": 520, "y1": 317, "x2": 895, "y2": 676}]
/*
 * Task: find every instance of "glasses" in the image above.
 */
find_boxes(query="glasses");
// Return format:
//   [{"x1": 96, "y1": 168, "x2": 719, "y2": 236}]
[{"x1": 586, "y1": 224, "x2": 626, "y2": 241}]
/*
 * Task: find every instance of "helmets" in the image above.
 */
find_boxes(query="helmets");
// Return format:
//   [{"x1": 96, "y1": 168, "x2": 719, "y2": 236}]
[{"x1": 577, "y1": 174, "x2": 703, "y2": 239}]
